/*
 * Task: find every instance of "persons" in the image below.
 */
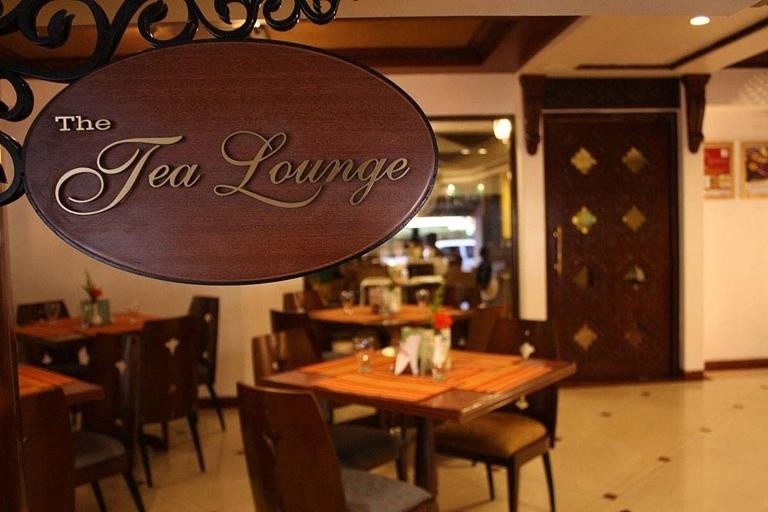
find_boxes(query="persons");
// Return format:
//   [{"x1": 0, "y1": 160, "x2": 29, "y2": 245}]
[
  {"x1": 477, "y1": 247, "x2": 490, "y2": 283},
  {"x1": 422, "y1": 233, "x2": 443, "y2": 260}
]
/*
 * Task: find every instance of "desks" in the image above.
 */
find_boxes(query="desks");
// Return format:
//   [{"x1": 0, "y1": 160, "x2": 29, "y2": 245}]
[
  {"x1": 14, "y1": 312, "x2": 158, "y2": 411},
  {"x1": 258, "y1": 345, "x2": 576, "y2": 508},
  {"x1": 14, "y1": 363, "x2": 103, "y2": 491},
  {"x1": 307, "y1": 305, "x2": 466, "y2": 416}
]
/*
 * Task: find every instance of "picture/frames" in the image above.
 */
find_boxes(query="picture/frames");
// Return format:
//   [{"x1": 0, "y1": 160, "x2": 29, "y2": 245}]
[{"x1": 703, "y1": 137, "x2": 766, "y2": 201}]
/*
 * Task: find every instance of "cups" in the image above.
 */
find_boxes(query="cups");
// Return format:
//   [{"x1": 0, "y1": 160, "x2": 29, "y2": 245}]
[{"x1": 339, "y1": 287, "x2": 433, "y2": 321}]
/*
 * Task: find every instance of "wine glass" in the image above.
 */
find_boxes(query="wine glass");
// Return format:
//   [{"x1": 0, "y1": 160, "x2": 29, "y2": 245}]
[
  {"x1": 351, "y1": 331, "x2": 451, "y2": 385},
  {"x1": 42, "y1": 299, "x2": 142, "y2": 330}
]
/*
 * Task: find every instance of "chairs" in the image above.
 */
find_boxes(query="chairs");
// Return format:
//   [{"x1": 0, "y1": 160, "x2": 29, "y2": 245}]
[
  {"x1": 159, "y1": 296, "x2": 218, "y2": 438},
  {"x1": 271, "y1": 265, "x2": 518, "y2": 352},
  {"x1": 105, "y1": 316, "x2": 205, "y2": 487},
  {"x1": 12, "y1": 386, "x2": 76, "y2": 510},
  {"x1": 236, "y1": 381, "x2": 434, "y2": 512},
  {"x1": 76, "y1": 330, "x2": 145, "y2": 510},
  {"x1": 433, "y1": 318, "x2": 558, "y2": 510},
  {"x1": 250, "y1": 328, "x2": 341, "y2": 466},
  {"x1": 18, "y1": 300, "x2": 92, "y2": 376}
]
